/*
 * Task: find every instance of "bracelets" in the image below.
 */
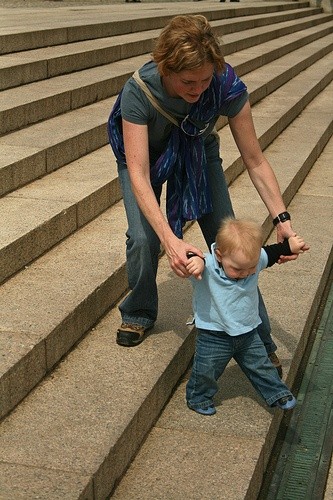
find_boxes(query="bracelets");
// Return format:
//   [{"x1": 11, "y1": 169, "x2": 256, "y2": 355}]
[{"x1": 272, "y1": 211, "x2": 291, "y2": 225}]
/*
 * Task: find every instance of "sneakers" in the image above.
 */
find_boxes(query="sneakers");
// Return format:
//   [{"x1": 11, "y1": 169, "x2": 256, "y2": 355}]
[
  {"x1": 269, "y1": 353, "x2": 282, "y2": 379},
  {"x1": 276, "y1": 395, "x2": 297, "y2": 411},
  {"x1": 116, "y1": 323, "x2": 154, "y2": 347}
]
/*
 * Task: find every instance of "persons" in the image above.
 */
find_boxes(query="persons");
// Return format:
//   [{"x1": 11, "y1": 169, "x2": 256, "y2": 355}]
[
  {"x1": 185, "y1": 217, "x2": 304, "y2": 415},
  {"x1": 106, "y1": 15, "x2": 310, "y2": 347}
]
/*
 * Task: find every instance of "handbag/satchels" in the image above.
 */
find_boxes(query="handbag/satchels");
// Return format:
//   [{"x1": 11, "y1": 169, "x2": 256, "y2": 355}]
[{"x1": 210, "y1": 126, "x2": 220, "y2": 148}]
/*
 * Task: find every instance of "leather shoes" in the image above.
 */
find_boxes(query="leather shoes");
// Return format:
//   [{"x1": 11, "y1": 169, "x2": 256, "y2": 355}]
[{"x1": 194, "y1": 405, "x2": 217, "y2": 416}]
